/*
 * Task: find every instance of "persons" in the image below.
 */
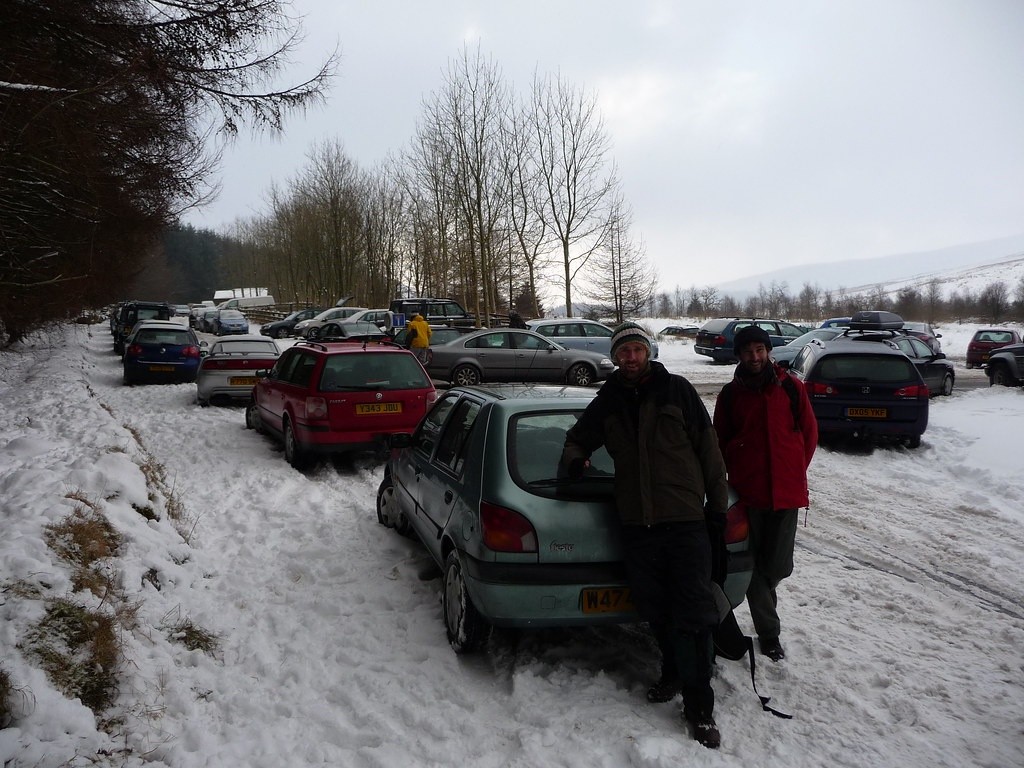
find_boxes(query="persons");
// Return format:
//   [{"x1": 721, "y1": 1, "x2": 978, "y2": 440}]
[
  {"x1": 405, "y1": 315, "x2": 432, "y2": 369},
  {"x1": 556, "y1": 322, "x2": 729, "y2": 749},
  {"x1": 509, "y1": 310, "x2": 528, "y2": 346},
  {"x1": 713, "y1": 326, "x2": 818, "y2": 662}
]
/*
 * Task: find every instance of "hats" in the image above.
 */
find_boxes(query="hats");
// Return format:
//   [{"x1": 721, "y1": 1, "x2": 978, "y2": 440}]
[
  {"x1": 508, "y1": 310, "x2": 518, "y2": 319},
  {"x1": 609, "y1": 323, "x2": 651, "y2": 365},
  {"x1": 733, "y1": 325, "x2": 772, "y2": 358},
  {"x1": 410, "y1": 311, "x2": 419, "y2": 320}
]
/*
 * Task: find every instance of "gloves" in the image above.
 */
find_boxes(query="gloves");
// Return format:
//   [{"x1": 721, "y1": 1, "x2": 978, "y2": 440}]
[
  {"x1": 701, "y1": 505, "x2": 729, "y2": 537},
  {"x1": 567, "y1": 454, "x2": 591, "y2": 479}
]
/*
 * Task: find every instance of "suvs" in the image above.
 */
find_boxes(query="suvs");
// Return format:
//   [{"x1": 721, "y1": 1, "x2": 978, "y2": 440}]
[
  {"x1": 776, "y1": 340, "x2": 929, "y2": 448},
  {"x1": 245, "y1": 340, "x2": 440, "y2": 474},
  {"x1": 694, "y1": 317, "x2": 805, "y2": 364},
  {"x1": 109, "y1": 299, "x2": 175, "y2": 354},
  {"x1": 389, "y1": 298, "x2": 496, "y2": 348},
  {"x1": 832, "y1": 311, "x2": 955, "y2": 398},
  {"x1": 984, "y1": 342, "x2": 1024, "y2": 388}
]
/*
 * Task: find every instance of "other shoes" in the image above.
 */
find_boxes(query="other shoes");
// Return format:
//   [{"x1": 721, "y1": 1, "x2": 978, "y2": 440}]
[
  {"x1": 646, "y1": 674, "x2": 682, "y2": 702},
  {"x1": 760, "y1": 635, "x2": 783, "y2": 662},
  {"x1": 684, "y1": 712, "x2": 720, "y2": 748}
]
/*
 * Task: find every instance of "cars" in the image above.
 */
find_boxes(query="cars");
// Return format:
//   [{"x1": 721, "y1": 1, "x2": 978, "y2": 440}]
[
  {"x1": 170, "y1": 303, "x2": 219, "y2": 334},
  {"x1": 902, "y1": 321, "x2": 943, "y2": 356},
  {"x1": 293, "y1": 307, "x2": 369, "y2": 342},
  {"x1": 123, "y1": 319, "x2": 184, "y2": 362},
  {"x1": 818, "y1": 318, "x2": 852, "y2": 331},
  {"x1": 660, "y1": 325, "x2": 698, "y2": 338},
  {"x1": 491, "y1": 319, "x2": 659, "y2": 366},
  {"x1": 334, "y1": 309, "x2": 389, "y2": 339},
  {"x1": 195, "y1": 335, "x2": 295, "y2": 407},
  {"x1": 204, "y1": 309, "x2": 249, "y2": 336},
  {"x1": 768, "y1": 327, "x2": 850, "y2": 375},
  {"x1": 419, "y1": 328, "x2": 615, "y2": 388},
  {"x1": 122, "y1": 325, "x2": 209, "y2": 386},
  {"x1": 965, "y1": 328, "x2": 1024, "y2": 369},
  {"x1": 375, "y1": 385, "x2": 755, "y2": 655},
  {"x1": 259, "y1": 307, "x2": 331, "y2": 339}
]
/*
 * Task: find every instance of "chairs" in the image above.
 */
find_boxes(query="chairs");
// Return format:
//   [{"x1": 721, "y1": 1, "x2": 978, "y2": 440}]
[
  {"x1": 501, "y1": 337, "x2": 507, "y2": 347},
  {"x1": 574, "y1": 327, "x2": 580, "y2": 335},
  {"x1": 982, "y1": 335, "x2": 991, "y2": 341},
  {"x1": 152, "y1": 316, "x2": 159, "y2": 320},
  {"x1": 541, "y1": 427, "x2": 566, "y2": 445},
  {"x1": 524, "y1": 441, "x2": 562, "y2": 481},
  {"x1": 175, "y1": 334, "x2": 188, "y2": 344},
  {"x1": 144, "y1": 334, "x2": 157, "y2": 341},
  {"x1": 1002, "y1": 335, "x2": 1010, "y2": 341},
  {"x1": 129, "y1": 314, "x2": 133, "y2": 322},
  {"x1": 736, "y1": 327, "x2": 741, "y2": 332},
  {"x1": 766, "y1": 330, "x2": 773, "y2": 335},
  {"x1": 298, "y1": 364, "x2": 314, "y2": 387},
  {"x1": 349, "y1": 360, "x2": 373, "y2": 387}
]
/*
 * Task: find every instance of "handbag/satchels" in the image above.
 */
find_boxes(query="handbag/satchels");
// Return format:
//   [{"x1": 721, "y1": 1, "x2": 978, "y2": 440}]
[{"x1": 705, "y1": 580, "x2": 747, "y2": 661}]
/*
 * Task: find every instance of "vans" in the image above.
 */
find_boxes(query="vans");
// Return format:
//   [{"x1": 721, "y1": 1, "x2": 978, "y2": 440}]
[{"x1": 218, "y1": 295, "x2": 276, "y2": 312}]
